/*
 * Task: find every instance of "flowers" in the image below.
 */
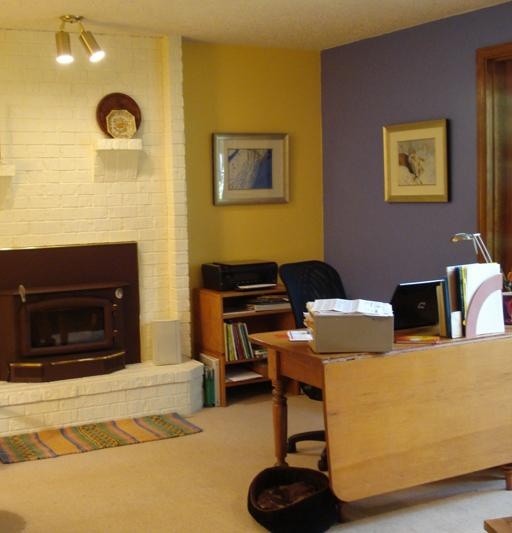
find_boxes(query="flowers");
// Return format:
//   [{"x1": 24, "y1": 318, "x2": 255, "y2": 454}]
[{"x1": 502, "y1": 270, "x2": 512, "y2": 291}]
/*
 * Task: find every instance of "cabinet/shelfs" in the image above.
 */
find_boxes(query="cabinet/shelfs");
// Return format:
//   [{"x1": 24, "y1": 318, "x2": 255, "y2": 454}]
[{"x1": 192, "y1": 283, "x2": 302, "y2": 407}]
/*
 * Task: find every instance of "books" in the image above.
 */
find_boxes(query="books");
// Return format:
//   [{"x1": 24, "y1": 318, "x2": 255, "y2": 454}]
[
  {"x1": 245, "y1": 295, "x2": 290, "y2": 311},
  {"x1": 223, "y1": 323, "x2": 255, "y2": 360},
  {"x1": 436, "y1": 261, "x2": 505, "y2": 339}
]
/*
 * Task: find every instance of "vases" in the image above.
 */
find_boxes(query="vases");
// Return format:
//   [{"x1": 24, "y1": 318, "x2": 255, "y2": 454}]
[{"x1": 502, "y1": 292, "x2": 512, "y2": 324}]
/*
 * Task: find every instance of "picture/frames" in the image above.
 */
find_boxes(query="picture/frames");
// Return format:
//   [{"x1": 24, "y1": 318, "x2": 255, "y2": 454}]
[
  {"x1": 212, "y1": 134, "x2": 289, "y2": 205},
  {"x1": 382, "y1": 118, "x2": 448, "y2": 204}
]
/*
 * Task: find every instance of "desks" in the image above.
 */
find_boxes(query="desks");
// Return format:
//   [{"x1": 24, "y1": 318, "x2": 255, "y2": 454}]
[{"x1": 247, "y1": 320, "x2": 512, "y2": 502}]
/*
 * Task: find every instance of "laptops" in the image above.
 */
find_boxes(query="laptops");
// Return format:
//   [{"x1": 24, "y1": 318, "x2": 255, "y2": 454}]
[{"x1": 389, "y1": 277, "x2": 444, "y2": 331}]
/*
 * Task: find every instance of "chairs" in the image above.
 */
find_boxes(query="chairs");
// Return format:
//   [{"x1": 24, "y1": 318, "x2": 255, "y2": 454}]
[{"x1": 278, "y1": 261, "x2": 346, "y2": 470}]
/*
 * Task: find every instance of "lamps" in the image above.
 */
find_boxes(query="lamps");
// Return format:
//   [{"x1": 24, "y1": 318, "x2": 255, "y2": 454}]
[
  {"x1": 51, "y1": 12, "x2": 106, "y2": 66},
  {"x1": 452, "y1": 230, "x2": 492, "y2": 265}
]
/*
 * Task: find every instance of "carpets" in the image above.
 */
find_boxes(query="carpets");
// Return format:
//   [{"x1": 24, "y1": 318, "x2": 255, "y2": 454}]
[{"x1": 0, "y1": 411, "x2": 203, "y2": 466}]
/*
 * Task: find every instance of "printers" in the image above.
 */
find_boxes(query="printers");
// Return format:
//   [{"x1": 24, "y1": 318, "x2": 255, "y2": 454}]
[{"x1": 201, "y1": 257, "x2": 278, "y2": 290}]
